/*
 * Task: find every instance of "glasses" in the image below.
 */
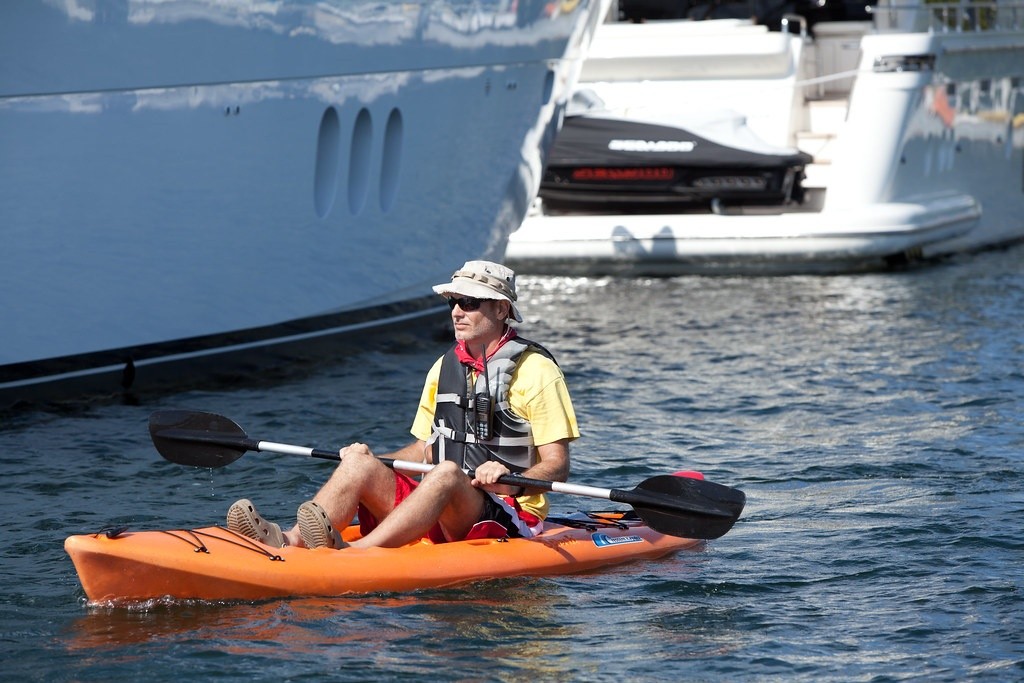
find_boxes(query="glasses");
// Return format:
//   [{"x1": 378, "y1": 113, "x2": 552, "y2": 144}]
[{"x1": 448, "y1": 295, "x2": 497, "y2": 312}]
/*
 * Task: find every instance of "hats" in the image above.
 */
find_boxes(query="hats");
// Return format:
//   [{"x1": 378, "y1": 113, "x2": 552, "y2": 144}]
[{"x1": 431, "y1": 260, "x2": 524, "y2": 324}]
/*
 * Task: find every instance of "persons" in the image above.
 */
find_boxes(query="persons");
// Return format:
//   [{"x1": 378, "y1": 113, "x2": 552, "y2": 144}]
[{"x1": 222, "y1": 259, "x2": 585, "y2": 551}]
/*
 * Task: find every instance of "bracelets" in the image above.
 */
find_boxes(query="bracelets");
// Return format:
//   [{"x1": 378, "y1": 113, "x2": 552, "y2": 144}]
[{"x1": 509, "y1": 472, "x2": 526, "y2": 498}]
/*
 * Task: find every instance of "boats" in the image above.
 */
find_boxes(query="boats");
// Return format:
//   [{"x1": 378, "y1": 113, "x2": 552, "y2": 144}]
[
  {"x1": 64, "y1": 472, "x2": 708, "y2": 607},
  {"x1": 503, "y1": 0, "x2": 1024, "y2": 277},
  {"x1": 0, "y1": 0, "x2": 601, "y2": 401}
]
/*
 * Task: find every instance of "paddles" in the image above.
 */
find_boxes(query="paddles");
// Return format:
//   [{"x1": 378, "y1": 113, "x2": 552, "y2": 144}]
[{"x1": 146, "y1": 407, "x2": 748, "y2": 542}]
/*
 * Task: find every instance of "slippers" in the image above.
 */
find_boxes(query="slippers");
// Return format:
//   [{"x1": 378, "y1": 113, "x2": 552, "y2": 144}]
[
  {"x1": 297, "y1": 501, "x2": 344, "y2": 551},
  {"x1": 226, "y1": 498, "x2": 287, "y2": 548}
]
